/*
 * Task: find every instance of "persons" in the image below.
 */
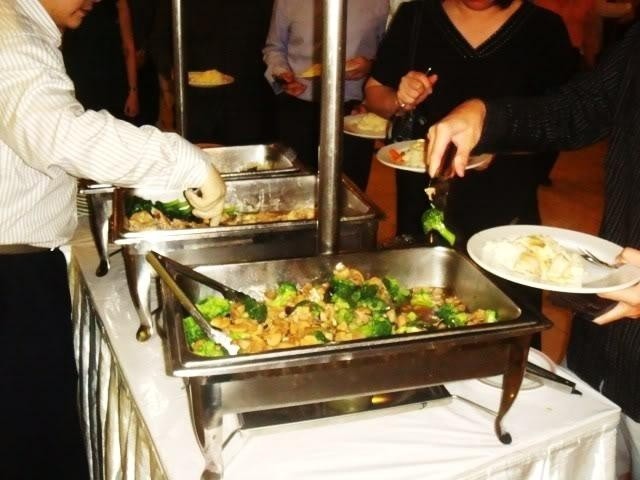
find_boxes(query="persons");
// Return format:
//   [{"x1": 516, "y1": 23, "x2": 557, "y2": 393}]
[
  {"x1": 359, "y1": 0, "x2": 578, "y2": 352},
  {"x1": 421, "y1": 0, "x2": 640, "y2": 430},
  {"x1": 1, "y1": 0, "x2": 230, "y2": 480}
]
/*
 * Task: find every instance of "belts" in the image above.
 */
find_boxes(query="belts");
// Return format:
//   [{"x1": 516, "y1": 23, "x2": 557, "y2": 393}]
[{"x1": 0, "y1": 244, "x2": 49, "y2": 255}]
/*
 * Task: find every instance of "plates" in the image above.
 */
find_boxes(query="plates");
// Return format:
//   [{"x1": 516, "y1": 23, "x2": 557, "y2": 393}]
[
  {"x1": 377, "y1": 140, "x2": 487, "y2": 173},
  {"x1": 343, "y1": 112, "x2": 395, "y2": 141},
  {"x1": 478, "y1": 347, "x2": 558, "y2": 392},
  {"x1": 466, "y1": 224, "x2": 640, "y2": 293},
  {"x1": 296, "y1": 63, "x2": 360, "y2": 80},
  {"x1": 188, "y1": 71, "x2": 236, "y2": 89}
]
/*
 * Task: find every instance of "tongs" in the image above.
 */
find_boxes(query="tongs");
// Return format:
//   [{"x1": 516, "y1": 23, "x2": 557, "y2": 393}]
[
  {"x1": 509, "y1": 358, "x2": 583, "y2": 399},
  {"x1": 429, "y1": 141, "x2": 459, "y2": 213},
  {"x1": 146, "y1": 250, "x2": 267, "y2": 357}
]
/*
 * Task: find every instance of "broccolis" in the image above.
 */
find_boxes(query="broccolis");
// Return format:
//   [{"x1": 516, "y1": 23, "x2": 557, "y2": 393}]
[
  {"x1": 419, "y1": 208, "x2": 456, "y2": 243},
  {"x1": 126, "y1": 197, "x2": 192, "y2": 219},
  {"x1": 181, "y1": 275, "x2": 499, "y2": 358}
]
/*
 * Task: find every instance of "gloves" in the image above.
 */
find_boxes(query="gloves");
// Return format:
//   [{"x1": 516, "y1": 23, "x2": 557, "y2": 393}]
[{"x1": 185, "y1": 164, "x2": 225, "y2": 227}]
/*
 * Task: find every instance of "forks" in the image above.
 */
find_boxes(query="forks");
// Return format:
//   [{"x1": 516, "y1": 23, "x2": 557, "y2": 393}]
[{"x1": 576, "y1": 247, "x2": 626, "y2": 270}]
